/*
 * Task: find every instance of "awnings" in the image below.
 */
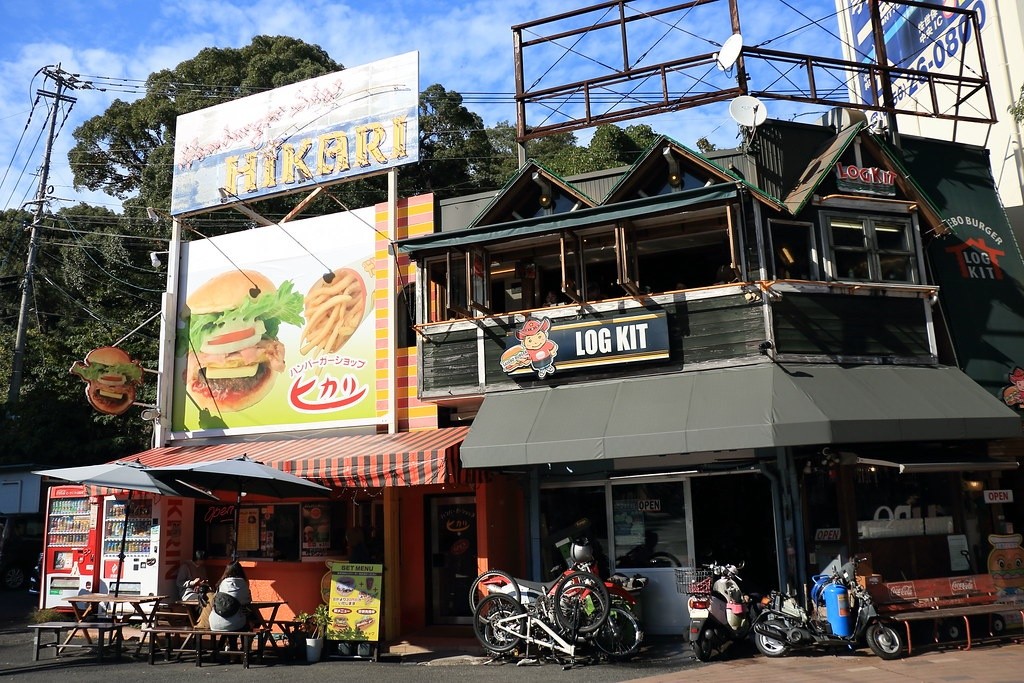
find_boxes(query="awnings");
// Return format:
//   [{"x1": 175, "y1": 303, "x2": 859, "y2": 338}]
[
  {"x1": 459, "y1": 364, "x2": 1024, "y2": 469},
  {"x1": 103, "y1": 425, "x2": 497, "y2": 488}
]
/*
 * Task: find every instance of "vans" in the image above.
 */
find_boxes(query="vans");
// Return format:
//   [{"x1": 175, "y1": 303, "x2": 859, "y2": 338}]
[{"x1": 0, "y1": 512, "x2": 45, "y2": 590}]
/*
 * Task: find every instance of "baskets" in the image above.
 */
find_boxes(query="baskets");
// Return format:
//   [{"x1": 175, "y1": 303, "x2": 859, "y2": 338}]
[{"x1": 674, "y1": 568, "x2": 714, "y2": 594}]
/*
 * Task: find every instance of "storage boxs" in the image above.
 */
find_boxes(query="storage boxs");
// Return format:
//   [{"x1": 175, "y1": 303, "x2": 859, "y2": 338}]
[{"x1": 855, "y1": 572, "x2": 881, "y2": 599}]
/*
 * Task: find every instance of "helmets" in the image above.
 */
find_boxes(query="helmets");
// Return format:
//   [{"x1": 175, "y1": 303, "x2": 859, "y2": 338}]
[{"x1": 570, "y1": 538, "x2": 593, "y2": 563}]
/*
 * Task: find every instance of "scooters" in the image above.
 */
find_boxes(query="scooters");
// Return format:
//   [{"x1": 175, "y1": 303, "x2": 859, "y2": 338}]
[{"x1": 674, "y1": 560, "x2": 750, "y2": 661}]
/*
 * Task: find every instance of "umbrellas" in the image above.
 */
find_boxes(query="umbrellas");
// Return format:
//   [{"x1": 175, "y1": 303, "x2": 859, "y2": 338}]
[
  {"x1": 140, "y1": 451, "x2": 332, "y2": 563},
  {"x1": 31, "y1": 455, "x2": 220, "y2": 653}
]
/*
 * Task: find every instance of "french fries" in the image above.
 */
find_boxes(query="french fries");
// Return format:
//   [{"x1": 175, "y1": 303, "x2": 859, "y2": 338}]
[{"x1": 299, "y1": 270, "x2": 363, "y2": 360}]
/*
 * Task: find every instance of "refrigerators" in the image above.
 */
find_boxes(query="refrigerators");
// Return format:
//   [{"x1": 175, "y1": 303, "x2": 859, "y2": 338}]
[
  {"x1": 39, "y1": 485, "x2": 103, "y2": 613},
  {"x1": 98, "y1": 494, "x2": 193, "y2": 621}
]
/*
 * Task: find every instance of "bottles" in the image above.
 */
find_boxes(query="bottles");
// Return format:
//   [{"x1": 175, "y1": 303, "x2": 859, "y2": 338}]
[
  {"x1": 51, "y1": 518, "x2": 60, "y2": 532},
  {"x1": 64, "y1": 534, "x2": 75, "y2": 545},
  {"x1": 71, "y1": 520, "x2": 89, "y2": 532},
  {"x1": 61, "y1": 517, "x2": 71, "y2": 532},
  {"x1": 49, "y1": 535, "x2": 63, "y2": 545},
  {"x1": 140, "y1": 542, "x2": 149, "y2": 551},
  {"x1": 124, "y1": 542, "x2": 139, "y2": 552},
  {"x1": 53, "y1": 501, "x2": 71, "y2": 514},
  {"x1": 71, "y1": 500, "x2": 90, "y2": 514},
  {"x1": 76, "y1": 534, "x2": 88, "y2": 545},
  {"x1": 849, "y1": 268, "x2": 853, "y2": 279},
  {"x1": 107, "y1": 541, "x2": 122, "y2": 552},
  {"x1": 127, "y1": 522, "x2": 151, "y2": 536},
  {"x1": 108, "y1": 523, "x2": 123, "y2": 537}
]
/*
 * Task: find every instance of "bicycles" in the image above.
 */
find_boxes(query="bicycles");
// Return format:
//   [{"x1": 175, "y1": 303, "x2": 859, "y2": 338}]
[{"x1": 469, "y1": 570, "x2": 645, "y2": 671}]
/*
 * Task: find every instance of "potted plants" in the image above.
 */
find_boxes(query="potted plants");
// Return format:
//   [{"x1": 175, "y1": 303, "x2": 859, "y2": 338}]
[{"x1": 292, "y1": 605, "x2": 332, "y2": 662}]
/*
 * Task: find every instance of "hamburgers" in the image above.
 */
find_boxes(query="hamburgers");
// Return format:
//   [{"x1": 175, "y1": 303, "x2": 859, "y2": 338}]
[
  {"x1": 175, "y1": 268, "x2": 305, "y2": 411},
  {"x1": 70, "y1": 347, "x2": 143, "y2": 416}
]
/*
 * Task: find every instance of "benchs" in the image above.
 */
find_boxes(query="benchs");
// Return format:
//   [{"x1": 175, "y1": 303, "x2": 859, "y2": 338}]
[
  {"x1": 869, "y1": 574, "x2": 1024, "y2": 654},
  {"x1": 191, "y1": 620, "x2": 303, "y2": 652},
  {"x1": 94, "y1": 612, "x2": 189, "y2": 648},
  {"x1": 141, "y1": 627, "x2": 273, "y2": 669},
  {"x1": 27, "y1": 622, "x2": 129, "y2": 660}
]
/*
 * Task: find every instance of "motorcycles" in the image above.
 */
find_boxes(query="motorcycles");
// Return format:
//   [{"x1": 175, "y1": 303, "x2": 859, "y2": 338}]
[
  {"x1": 478, "y1": 520, "x2": 644, "y2": 660},
  {"x1": 748, "y1": 557, "x2": 903, "y2": 661}
]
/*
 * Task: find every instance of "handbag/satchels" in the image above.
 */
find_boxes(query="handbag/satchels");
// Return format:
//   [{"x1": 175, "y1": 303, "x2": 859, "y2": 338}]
[{"x1": 214, "y1": 586, "x2": 244, "y2": 618}]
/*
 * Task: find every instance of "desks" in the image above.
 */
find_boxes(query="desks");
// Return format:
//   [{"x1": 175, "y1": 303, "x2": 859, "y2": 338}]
[
  {"x1": 59, "y1": 593, "x2": 170, "y2": 659},
  {"x1": 160, "y1": 599, "x2": 288, "y2": 665}
]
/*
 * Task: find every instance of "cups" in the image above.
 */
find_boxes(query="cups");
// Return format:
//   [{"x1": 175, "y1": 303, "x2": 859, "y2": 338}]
[{"x1": 366, "y1": 578, "x2": 373, "y2": 589}]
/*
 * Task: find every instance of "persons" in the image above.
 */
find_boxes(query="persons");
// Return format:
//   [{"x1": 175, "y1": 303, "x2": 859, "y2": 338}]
[
  {"x1": 176, "y1": 548, "x2": 214, "y2": 617},
  {"x1": 208, "y1": 560, "x2": 263, "y2": 660}
]
